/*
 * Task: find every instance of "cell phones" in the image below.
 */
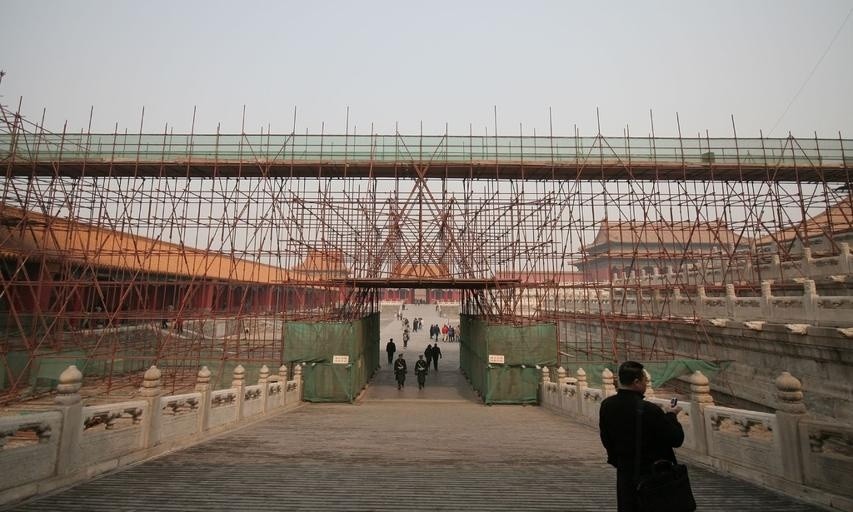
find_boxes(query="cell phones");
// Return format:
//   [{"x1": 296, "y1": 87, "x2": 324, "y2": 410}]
[{"x1": 671, "y1": 397, "x2": 676, "y2": 408}]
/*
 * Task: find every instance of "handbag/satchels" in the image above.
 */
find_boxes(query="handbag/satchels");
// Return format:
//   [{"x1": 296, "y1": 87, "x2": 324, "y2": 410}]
[{"x1": 634, "y1": 463, "x2": 697, "y2": 511}]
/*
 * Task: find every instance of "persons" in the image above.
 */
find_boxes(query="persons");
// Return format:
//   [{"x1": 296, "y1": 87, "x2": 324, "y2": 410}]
[
  {"x1": 597, "y1": 360, "x2": 685, "y2": 511},
  {"x1": 431, "y1": 343, "x2": 442, "y2": 370},
  {"x1": 423, "y1": 344, "x2": 432, "y2": 367},
  {"x1": 77, "y1": 297, "x2": 127, "y2": 328},
  {"x1": 175, "y1": 313, "x2": 183, "y2": 334},
  {"x1": 413, "y1": 354, "x2": 428, "y2": 390},
  {"x1": 385, "y1": 338, "x2": 395, "y2": 364},
  {"x1": 402, "y1": 297, "x2": 427, "y2": 311},
  {"x1": 396, "y1": 311, "x2": 460, "y2": 342},
  {"x1": 160, "y1": 319, "x2": 167, "y2": 329},
  {"x1": 392, "y1": 353, "x2": 407, "y2": 390},
  {"x1": 402, "y1": 330, "x2": 409, "y2": 347}
]
